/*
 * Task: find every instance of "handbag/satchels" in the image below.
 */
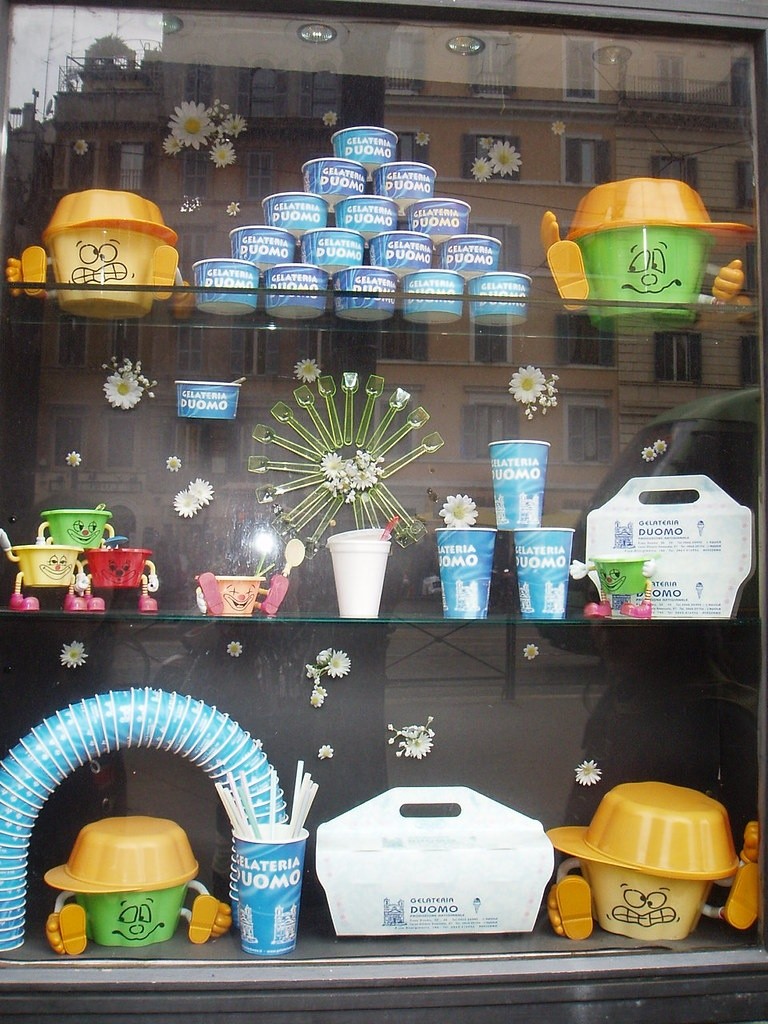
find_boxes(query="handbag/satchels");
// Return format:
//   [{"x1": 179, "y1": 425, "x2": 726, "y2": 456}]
[
  {"x1": 584, "y1": 473, "x2": 755, "y2": 618},
  {"x1": 314, "y1": 785, "x2": 556, "y2": 936}
]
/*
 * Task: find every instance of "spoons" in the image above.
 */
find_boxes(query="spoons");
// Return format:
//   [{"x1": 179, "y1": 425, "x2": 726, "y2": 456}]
[
  {"x1": 281, "y1": 539, "x2": 305, "y2": 577},
  {"x1": 248, "y1": 373, "x2": 444, "y2": 559}
]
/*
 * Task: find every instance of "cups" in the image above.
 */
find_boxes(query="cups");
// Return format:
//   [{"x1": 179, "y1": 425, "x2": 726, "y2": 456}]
[
  {"x1": 0, "y1": 687, "x2": 310, "y2": 955},
  {"x1": 514, "y1": 527, "x2": 576, "y2": 621},
  {"x1": 435, "y1": 528, "x2": 498, "y2": 620},
  {"x1": 487, "y1": 439, "x2": 552, "y2": 532},
  {"x1": 326, "y1": 528, "x2": 391, "y2": 619}
]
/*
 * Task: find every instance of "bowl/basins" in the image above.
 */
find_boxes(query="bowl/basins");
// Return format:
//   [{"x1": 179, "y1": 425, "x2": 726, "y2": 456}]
[
  {"x1": 191, "y1": 125, "x2": 533, "y2": 326},
  {"x1": 87, "y1": 549, "x2": 152, "y2": 589},
  {"x1": 40, "y1": 509, "x2": 113, "y2": 549},
  {"x1": 12, "y1": 545, "x2": 83, "y2": 587},
  {"x1": 588, "y1": 555, "x2": 660, "y2": 595},
  {"x1": 173, "y1": 380, "x2": 241, "y2": 420},
  {"x1": 195, "y1": 575, "x2": 266, "y2": 617}
]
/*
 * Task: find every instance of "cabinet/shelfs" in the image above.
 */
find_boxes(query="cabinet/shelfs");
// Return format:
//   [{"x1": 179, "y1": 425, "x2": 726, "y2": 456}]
[{"x1": 0, "y1": 1, "x2": 767, "y2": 1022}]
